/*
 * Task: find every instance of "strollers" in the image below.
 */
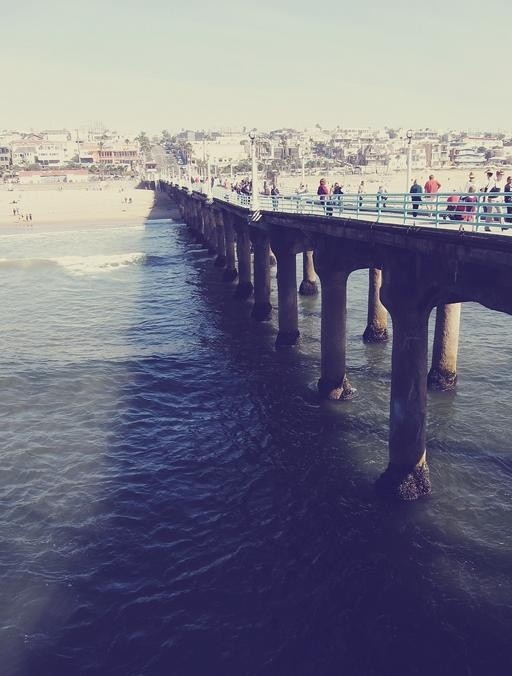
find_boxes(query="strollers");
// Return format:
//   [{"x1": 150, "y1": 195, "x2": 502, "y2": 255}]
[{"x1": 443, "y1": 196, "x2": 466, "y2": 220}]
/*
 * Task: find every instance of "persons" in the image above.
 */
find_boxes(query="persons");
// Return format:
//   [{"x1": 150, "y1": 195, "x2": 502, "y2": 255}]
[
  {"x1": 444, "y1": 170, "x2": 512, "y2": 231},
  {"x1": 295, "y1": 178, "x2": 344, "y2": 217},
  {"x1": 271, "y1": 184, "x2": 279, "y2": 210},
  {"x1": 376, "y1": 186, "x2": 388, "y2": 207},
  {"x1": 259, "y1": 180, "x2": 271, "y2": 196},
  {"x1": 180, "y1": 171, "x2": 252, "y2": 205},
  {"x1": 410, "y1": 179, "x2": 423, "y2": 218},
  {"x1": 424, "y1": 175, "x2": 441, "y2": 216},
  {"x1": 359, "y1": 180, "x2": 366, "y2": 206}
]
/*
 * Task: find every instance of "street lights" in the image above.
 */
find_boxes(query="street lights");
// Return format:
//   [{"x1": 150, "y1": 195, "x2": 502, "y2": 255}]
[
  {"x1": 300, "y1": 149, "x2": 305, "y2": 186},
  {"x1": 249, "y1": 129, "x2": 258, "y2": 210},
  {"x1": 403, "y1": 129, "x2": 414, "y2": 193}
]
